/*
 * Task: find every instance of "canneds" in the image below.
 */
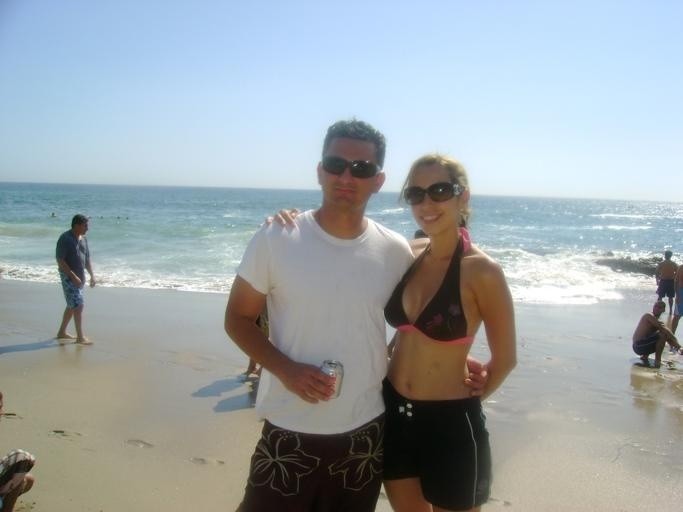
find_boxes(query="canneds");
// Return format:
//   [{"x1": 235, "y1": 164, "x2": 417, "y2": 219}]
[{"x1": 320, "y1": 359, "x2": 344, "y2": 399}]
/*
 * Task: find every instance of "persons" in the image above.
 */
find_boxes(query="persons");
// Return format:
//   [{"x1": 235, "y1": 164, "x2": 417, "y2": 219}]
[
  {"x1": 266, "y1": 155, "x2": 516, "y2": 511},
  {"x1": 631, "y1": 300, "x2": 682, "y2": 368},
  {"x1": 654, "y1": 250, "x2": 677, "y2": 316},
  {"x1": 668, "y1": 264, "x2": 683, "y2": 352},
  {"x1": 242, "y1": 316, "x2": 264, "y2": 381},
  {"x1": 55, "y1": 213, "x2": 95, "y2": 345},
  {"x1": 222, "y1": 118, "x2": 492, "y2": 512}
]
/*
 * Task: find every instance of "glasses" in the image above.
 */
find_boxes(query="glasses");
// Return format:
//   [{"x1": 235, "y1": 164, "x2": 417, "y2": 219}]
[
  {"x1": 321, "y1": 156, "x2": 379, "y2": 178},
  {"x1": 404, "y1": 182, "x2": 463, "y2": 205}
]
